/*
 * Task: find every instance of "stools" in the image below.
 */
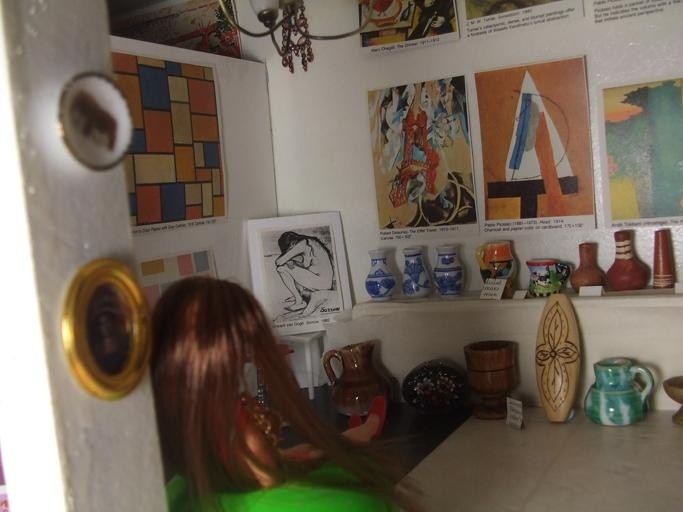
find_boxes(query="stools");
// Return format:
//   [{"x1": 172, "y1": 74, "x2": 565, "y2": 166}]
[{"x1": 282, "y1": 330, "x2": 327, "y2": 401}]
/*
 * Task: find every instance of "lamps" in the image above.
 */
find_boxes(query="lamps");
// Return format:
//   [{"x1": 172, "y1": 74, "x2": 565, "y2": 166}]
[{"x1": 218, "y1": 0, "x2": 373, "y2": 75}]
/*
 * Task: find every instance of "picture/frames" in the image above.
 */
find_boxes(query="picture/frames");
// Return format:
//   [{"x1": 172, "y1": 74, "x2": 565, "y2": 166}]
[
  {"x1": 60, "y1": 254, "x2": 151, "y2": 402},
  {"x1": 248, "y1": 209, "x2": 354, "y2": 338}
]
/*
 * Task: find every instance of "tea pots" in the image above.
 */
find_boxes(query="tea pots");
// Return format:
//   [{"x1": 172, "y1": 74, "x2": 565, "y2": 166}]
[{"x1": 323, "y1": 341, "x2": 392, "y2": 416}]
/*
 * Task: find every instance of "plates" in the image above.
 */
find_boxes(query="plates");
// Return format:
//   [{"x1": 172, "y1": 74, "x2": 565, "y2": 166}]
[{"x1": 57, "y1": 71, "x2": 134, "y2": 171}]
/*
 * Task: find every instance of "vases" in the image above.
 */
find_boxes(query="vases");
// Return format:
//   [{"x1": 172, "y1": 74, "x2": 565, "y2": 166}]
[
  {"x1": 571, "y1": 241, "x2": 604, "y2": 292},
  {"x1": 402, "y1": 244, "x2": 432, "y2": 299},
  {"x1": 432, "y1": 243, "x2": 468, "y2": 299},
  {"x1": 365, "y1": 248, "x2": 397, "y2": 301}
]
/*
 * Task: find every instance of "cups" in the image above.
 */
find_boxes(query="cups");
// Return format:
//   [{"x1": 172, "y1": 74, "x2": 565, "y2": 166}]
[
  {"x1": 527, "y1": 258, "x2": 570, "y2": 298},
  {"x1": 583, "y1": 356, "x2": 655, "y2": 427}
]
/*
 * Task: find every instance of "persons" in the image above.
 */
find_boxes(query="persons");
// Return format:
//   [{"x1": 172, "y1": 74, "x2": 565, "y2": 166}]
[
  {"x1": 151, "y1": 277, "x2": 428, "y2": 512},
  {"x1": 282, "y1": 394, "x2": 387, "y2": 462},
  {"x1": 274, "y1": 231, "x2": 333, "y2": 312}
]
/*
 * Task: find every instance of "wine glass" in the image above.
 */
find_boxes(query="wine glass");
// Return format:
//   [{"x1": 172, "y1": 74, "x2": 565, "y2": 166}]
[{"x1": 464, "y1": 340, "x2": 519, "y2": 418}]
